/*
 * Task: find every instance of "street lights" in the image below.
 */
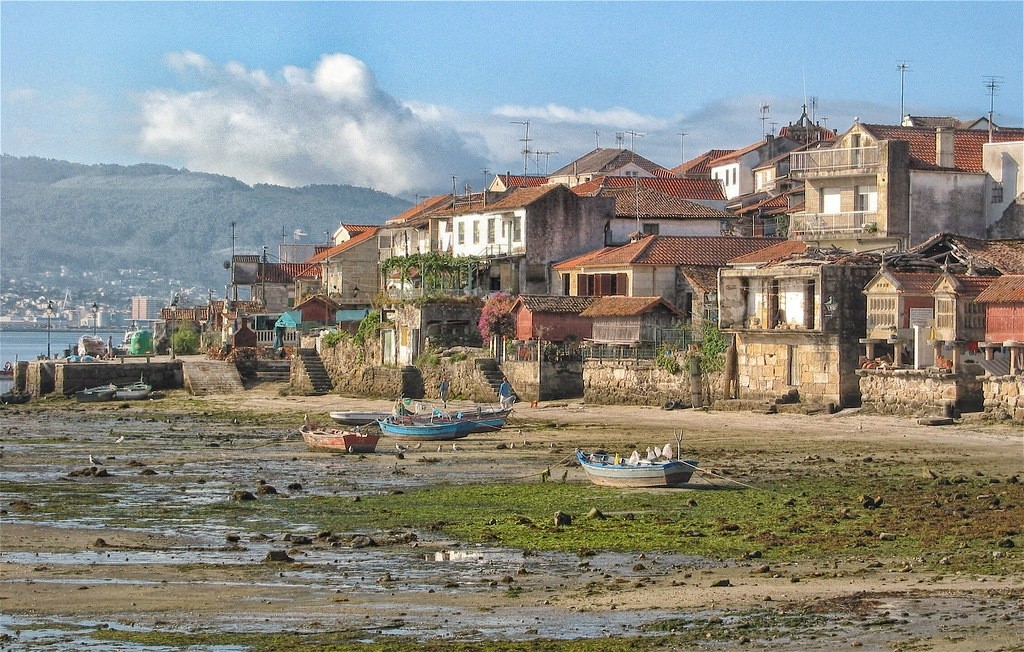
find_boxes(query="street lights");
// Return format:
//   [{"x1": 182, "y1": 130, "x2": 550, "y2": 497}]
[
  {"x1": 46, "y1": 303, "x2": 53, "y2": 363},
  {"x1": 91, "y1": 302, "x2": 100, "y2": 337}
]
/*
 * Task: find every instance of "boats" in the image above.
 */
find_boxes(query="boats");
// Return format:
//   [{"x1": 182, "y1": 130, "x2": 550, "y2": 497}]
[
  {"x1": 114, "y1": 380, "x2": 153, "y2": 400},
  {"x1": 329, "y1": 410, "x2": 394, "y2": 426},
  {"x1": 573, "y1": 446, "x2": 698, "y2": 486},
  {"x1": 123, "y1": 318, "x2": 141, "y2": 344},
  {"x1": 0, "y1": 367, "x2": 14, "y2": 377},
  {"x1": 75, "y1": 383, "x2": 118, "y2": 402},
  {"x1": 298, "y1": 413, "x2": 380, "y2": 455},
  {"x1": 383, "y1": 404, "x2": 517, "y2": 438},
  {"x1": 374, "y1": 418, "x2": 462, "y2": 441}
]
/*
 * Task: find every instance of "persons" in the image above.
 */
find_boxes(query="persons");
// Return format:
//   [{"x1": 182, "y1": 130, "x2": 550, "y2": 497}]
[
  {"x1": 395, "y1": 399, "x2": 414, "y2": 415},
  {"x1": 439, "y1": 376, "x2": 448, "y2": 409},
  {"x1": 499, "y1": 377, "x2": 510, "y2": 409},
  {"x1": 103, "y1": 336, "x2": 113, "y2": 361}
]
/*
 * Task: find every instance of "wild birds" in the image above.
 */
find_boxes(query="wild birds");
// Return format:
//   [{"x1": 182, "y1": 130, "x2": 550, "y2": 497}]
[
  {"x1": 114, "y1": 436, "x2": 125, "y2": 445},
  {"x1": 395, "y1": 442, "x2": 465, "y2": 459},
  {"x1": 88, "y1": 453, "x2": 103, "y2": 467},
  {"x1": 348, "y1": 445, "x2": 354, "y2": 455}
]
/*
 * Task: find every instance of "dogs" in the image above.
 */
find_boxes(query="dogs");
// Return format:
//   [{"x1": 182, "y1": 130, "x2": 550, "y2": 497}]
[{"x1": 531, "y1": 400, "x2": 538, "y2": 410}]
